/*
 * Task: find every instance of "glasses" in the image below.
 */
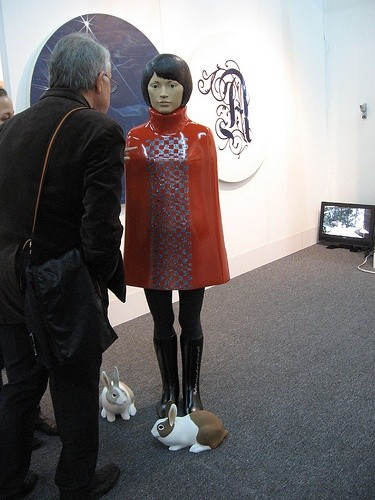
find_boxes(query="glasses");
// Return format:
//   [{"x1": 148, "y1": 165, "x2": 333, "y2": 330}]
[{"x1": 98, "y1": 71, "x2": 119, "y2": 94}]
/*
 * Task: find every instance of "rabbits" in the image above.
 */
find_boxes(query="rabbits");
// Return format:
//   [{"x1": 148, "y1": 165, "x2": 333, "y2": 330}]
[
  {"x1": 149, "y1": 400, "x2": 229, "y2": 454},
  {"x1": 100, "y1": 365, "x2": 137, "y2": 423}
]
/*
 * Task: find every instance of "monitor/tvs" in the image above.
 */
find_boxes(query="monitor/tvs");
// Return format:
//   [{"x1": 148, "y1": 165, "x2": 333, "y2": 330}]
[{"x1": 318, "y1": 202, "x2": 375, "y2": 248}]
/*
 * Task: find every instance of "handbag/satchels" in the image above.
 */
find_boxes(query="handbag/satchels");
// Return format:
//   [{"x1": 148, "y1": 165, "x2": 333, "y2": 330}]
[{"x1": 22, "y1": 246, "x2": 104, "y2": 373}]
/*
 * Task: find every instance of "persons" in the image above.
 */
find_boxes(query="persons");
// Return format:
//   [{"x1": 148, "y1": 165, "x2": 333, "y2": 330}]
[
  {"x1": 0, "y1": 32, "x2": 125, "y2": 499},
  {"x1": 121, "y1": 55, "x2": 230, "y2": 418}
]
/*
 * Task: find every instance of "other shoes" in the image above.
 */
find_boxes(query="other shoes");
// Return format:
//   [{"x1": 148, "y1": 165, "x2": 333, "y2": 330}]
[
  {"x1": 13, "y1": 469, "x2": 38, "y2": 500},
  {"x1": 59, "y1": 463, "x2": 120, "y2": 500},
  {"x1": 34, "y1": 407, "x2": 60, "y2": 435},
  {"x1": 30, "y1": 435, "x2": 46, "y2": 451}
]
